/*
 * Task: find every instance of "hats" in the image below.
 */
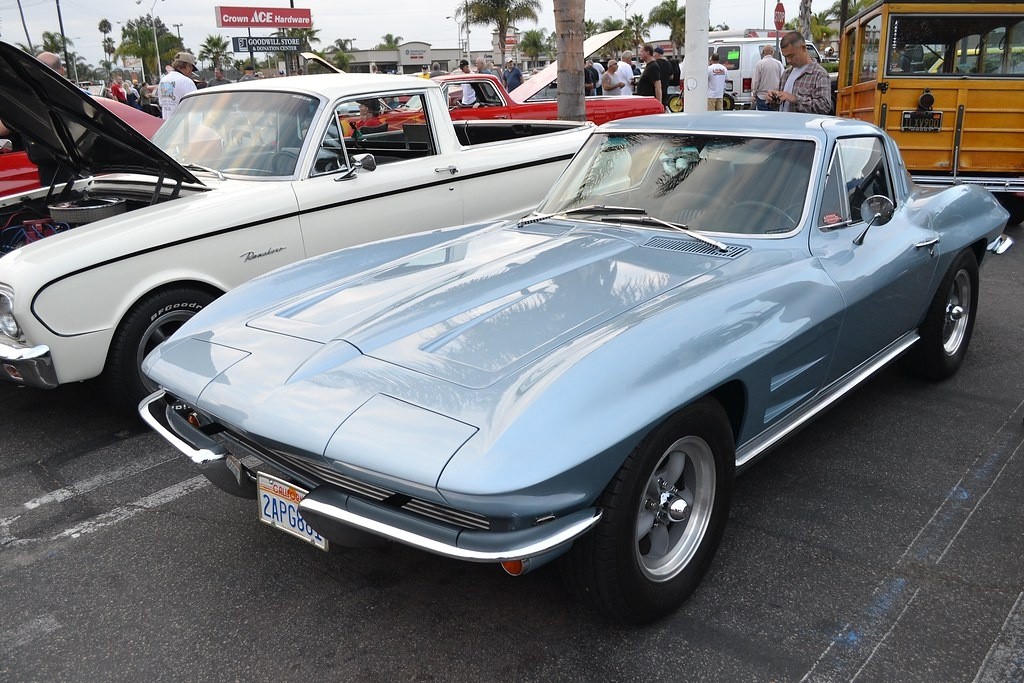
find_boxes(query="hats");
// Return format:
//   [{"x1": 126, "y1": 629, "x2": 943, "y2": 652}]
[
  {"x1": 421, "y1": 65, "x2": 427, "y2": 68},
  {"x1": 654, "y1": 48, "x2": 664, "y2": 54},
  {"x1": 173, "y1": 52, "x2": 197, "y2": 71},
  {"x1": 504, "y1": 57, "x2": 512, "y2": 64},
  {"x1": 458, "y1": 60, "x2": 468, "y2": 68}
]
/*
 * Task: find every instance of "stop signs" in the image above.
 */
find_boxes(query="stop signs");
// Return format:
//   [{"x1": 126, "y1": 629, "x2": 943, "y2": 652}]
[{"x1": 774, "y1": 3, "x2": 785, "y2": 30}]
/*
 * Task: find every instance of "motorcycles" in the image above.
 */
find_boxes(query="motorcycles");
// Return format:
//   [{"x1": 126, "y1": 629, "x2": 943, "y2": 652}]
[{"x1": 668, "y1": 79, "x2": 683, "y2": 113}]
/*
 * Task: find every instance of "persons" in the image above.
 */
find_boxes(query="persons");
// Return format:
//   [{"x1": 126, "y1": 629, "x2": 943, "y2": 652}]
[
  {"x1": 765, "y1": 31, "x2": 832, "y2": 115},
  {"x1": 0, "y1": 44, "x2": 683, "y2": 142},
  {"x1": 707, "y1": 53, "x2": 729, "y2": 111},
  {"x1": 750, "y1": 44, "x2": 785, "y2": 112}
]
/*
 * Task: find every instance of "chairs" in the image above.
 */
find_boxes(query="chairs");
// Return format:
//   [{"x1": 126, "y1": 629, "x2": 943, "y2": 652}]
[{"x1": 361, "y1": 122, "x2": 389, "y2": 135}]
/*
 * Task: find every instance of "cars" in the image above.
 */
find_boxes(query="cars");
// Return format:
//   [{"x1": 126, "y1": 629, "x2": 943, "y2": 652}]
[
  {"x1": 927, "y1": 47, "x2": 1024, "y2": 74},
  {"x1": 139, "y1": 111, "x2": 1013, "y2": 624},
  {"x1": 0, "y1": 96, "x2": 224, "y2": 199}
]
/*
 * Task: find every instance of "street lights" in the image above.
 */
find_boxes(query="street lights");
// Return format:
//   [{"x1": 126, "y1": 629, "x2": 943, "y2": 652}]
[
  {"x1": 446, "y1": 15, "x2": 471, "y2": 60},
  {"x1": 116, "y1": 19, "x2": 146, "y2": 82},
  {"x1": 135, "y1": 0, "x2": 165, "y2": 81},
  {"x1": 71, "y1": 37, "x2": 81, "y2": 87}
]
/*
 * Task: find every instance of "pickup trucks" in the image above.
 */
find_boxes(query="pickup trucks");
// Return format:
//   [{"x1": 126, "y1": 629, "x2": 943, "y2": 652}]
[
  {"x1": 0, "y1": 39, "x2": 632, "y2": 416},
  {"x1": 359, "y1": 74, "x2": 665, "y2": 127}
]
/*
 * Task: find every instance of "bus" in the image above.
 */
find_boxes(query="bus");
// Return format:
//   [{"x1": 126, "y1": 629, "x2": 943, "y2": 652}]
[{"x1": 825, "y1": 1, "x2": 1024, "y2": 225}]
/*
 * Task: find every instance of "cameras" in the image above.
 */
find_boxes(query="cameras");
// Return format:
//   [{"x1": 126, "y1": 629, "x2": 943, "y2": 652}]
[{"x1": 768, "y1": 94, "x2": 782, "y2": 110}]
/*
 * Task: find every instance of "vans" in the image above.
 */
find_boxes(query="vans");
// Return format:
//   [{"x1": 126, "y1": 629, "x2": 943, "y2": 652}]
[{"x1": 680, "y1": 36, "x2": 822, "y2": 111}]
